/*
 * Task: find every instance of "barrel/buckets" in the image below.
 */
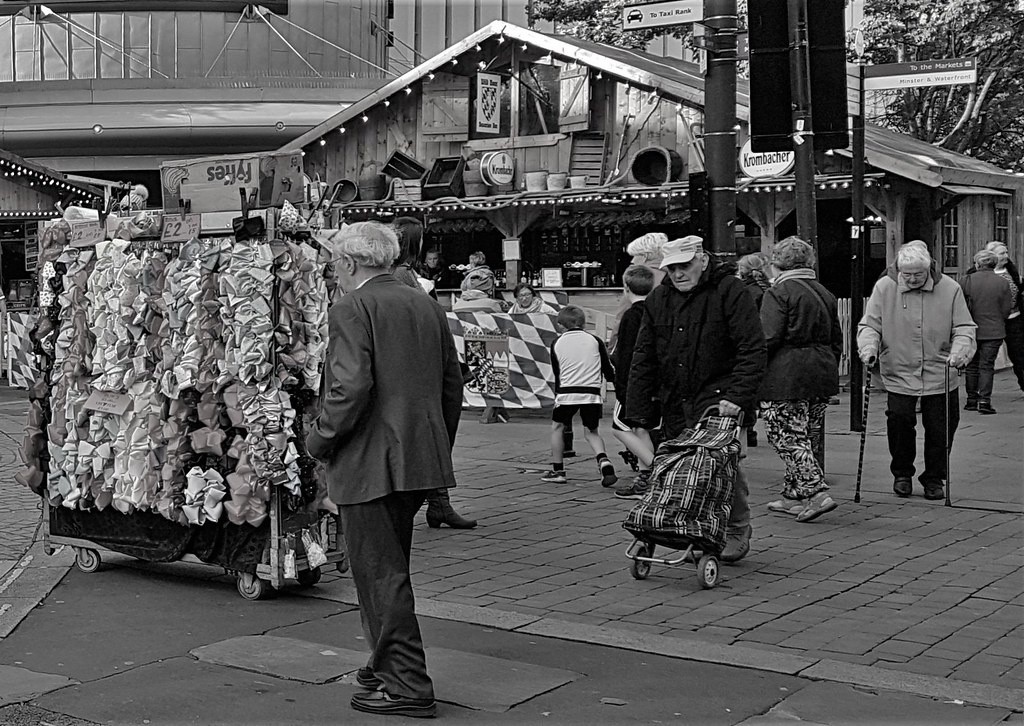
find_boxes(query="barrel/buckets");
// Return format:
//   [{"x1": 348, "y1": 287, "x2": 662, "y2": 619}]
[
  {"x1": 358, "y1": 174, "x2": 387, "y2": 201},
  {"x1": 463, "y1": 163, "x2": 488, "y2": 197},
  {"x1": 567, "y1": 174, "x2": 590, "y2": 188},
  {"x1": 543, "y1": 171, "x2": 568, "y2": 190},
  {"x1": 525, "y1": 170, "x2": 549, "y2": 191}
]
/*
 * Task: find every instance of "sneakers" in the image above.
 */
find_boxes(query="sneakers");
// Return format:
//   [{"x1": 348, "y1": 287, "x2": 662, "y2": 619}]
[
  {"x1": 597, "y1": 458, "x2": 618, "y2": 487},
  {"x1": 540, "y1": 471, "x2": 566, "y2": 482},
  {"x1": 614, "y1": 481, "x2": 648, "y2": 500}
]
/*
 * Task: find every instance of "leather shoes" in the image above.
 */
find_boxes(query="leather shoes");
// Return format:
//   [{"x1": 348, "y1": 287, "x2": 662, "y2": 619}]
[
  {"x1": 357, "y1": 667, "x2": 382, "y2": 685},
  {"x1": 924, "y1": 483, "x2": 944, "y2": 500},
  {"x1": 350, "y1": 688, "x2": 438, "y2": 716},
  {"x1": 893, "y1": 475, "x2": 912, "y2": 496}
]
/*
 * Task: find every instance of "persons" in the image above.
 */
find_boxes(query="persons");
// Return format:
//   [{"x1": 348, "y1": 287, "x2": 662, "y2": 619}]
[
  {"x1": 760, "y1": 235, "x2": 844, "y2": 523},
  {"x1": 0, "y1": 288, "x2": 7, "y2": 379},
  {"x1": 985, "y1": 241, "x2": 1024, "y2": 390},
  {"x1": 957, "y1": 250, "x2": 1011, "y2": 414},
  {"x1": 856, "y1": 240, "x2": 978, "y2": 501},
  {"x1": 392, "y1": 216, "x2": 776, "y2": 559},
  {"x1": 304, "y1": 222, "x2": 463, "y2": 719}
]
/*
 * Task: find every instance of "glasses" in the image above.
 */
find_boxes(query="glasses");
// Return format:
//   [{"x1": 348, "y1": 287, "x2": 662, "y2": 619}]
[
  {"x1": 518, "y1": 293, "x2": 533, "y2": 299},
  {"x1": 325, "y1": 257, "x2": 340, "y2": 272},
  {"x1": 901, "y1": 271, "x2": 923, "y2": 278}
]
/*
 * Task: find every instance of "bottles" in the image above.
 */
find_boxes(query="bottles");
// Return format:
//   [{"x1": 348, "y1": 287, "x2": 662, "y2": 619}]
[
  {"x1": 495, "y1": 270, "x2": 506, "y2": 288},
  {"x1": 521, "y1": 268, "x2": 542, "y2": 288}
]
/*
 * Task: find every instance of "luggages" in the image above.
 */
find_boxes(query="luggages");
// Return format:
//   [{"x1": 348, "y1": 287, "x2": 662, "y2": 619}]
[{"x1": 622, "y1": 404, "x2": 744, "y2": 589}]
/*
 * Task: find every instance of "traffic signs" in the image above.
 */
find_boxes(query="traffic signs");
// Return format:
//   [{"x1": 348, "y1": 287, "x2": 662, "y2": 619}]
[{"x1": 845, "y1": 57, "x2": 977, "y2": 115}]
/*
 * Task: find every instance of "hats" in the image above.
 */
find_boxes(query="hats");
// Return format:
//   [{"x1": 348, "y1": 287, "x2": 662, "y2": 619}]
[{"x1": 659, "y1": 235, "x2": 704, "y2": 269}]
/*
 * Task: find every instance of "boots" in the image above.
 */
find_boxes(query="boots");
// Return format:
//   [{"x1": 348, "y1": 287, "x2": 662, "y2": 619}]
[{"x1": 426, "y1": 487, "x2": 477, "y2": 529}]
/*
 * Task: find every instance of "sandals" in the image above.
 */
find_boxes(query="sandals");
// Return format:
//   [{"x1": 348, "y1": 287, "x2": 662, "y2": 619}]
[
  {"x1": 767, "y1": 497, "x2": 804, "y2": 515},
  {"x1": 795, "y1": 491, "x2": 838, "y2": 521}
]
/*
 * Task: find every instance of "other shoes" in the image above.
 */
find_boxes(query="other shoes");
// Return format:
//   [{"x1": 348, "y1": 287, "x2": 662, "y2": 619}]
[
  {"x1": 684, "y1": 549, "x2": 702, "y2": 561},
  {"x1": 720, "y1": 524, "x2": 752, "y2": 562},
  {"x1": 978, "y1": 403, "x2": 996, "y2": 413},
  {"x1": 964, "y1": 402, "x2": 977, "y2": 411}
]
